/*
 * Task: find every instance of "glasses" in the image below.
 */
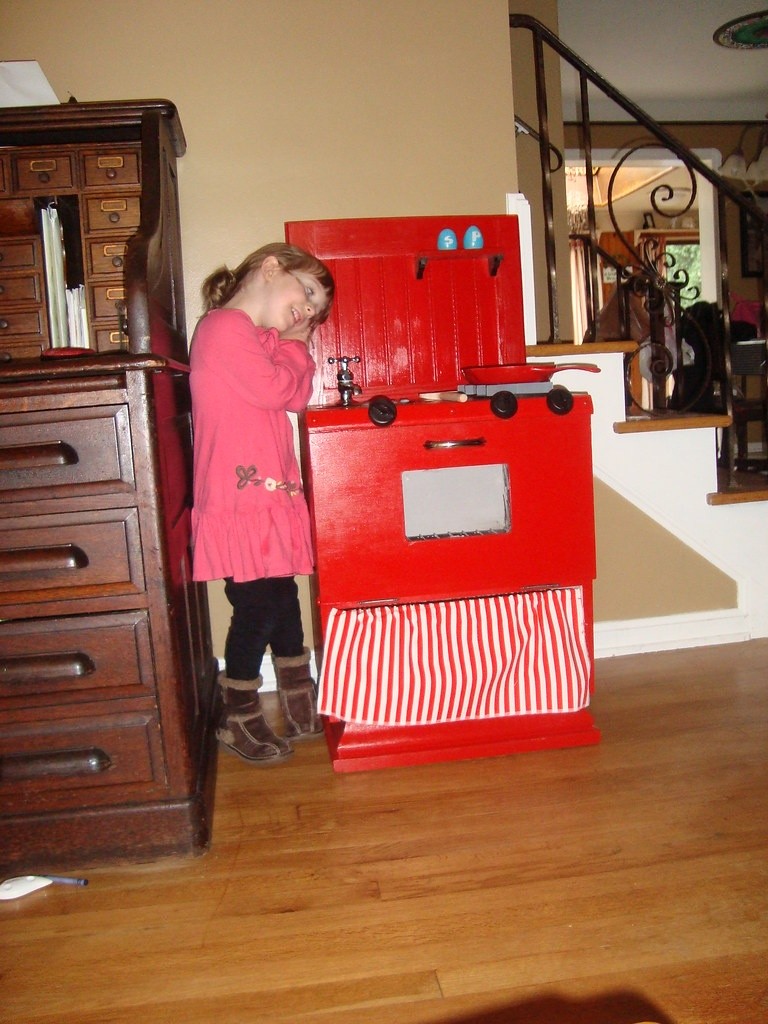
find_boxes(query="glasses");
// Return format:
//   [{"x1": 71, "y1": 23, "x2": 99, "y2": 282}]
[{"x1": 279, "y1": 259, "x2": 324, "y2": 321}]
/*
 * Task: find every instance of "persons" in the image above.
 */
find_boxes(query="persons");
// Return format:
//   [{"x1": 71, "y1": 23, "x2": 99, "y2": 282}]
[{"x1": 191, "y1": 242, "x2": 335, "y2": 761}]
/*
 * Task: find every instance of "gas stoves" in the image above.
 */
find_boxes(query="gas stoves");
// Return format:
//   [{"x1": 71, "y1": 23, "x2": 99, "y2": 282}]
[{"x1": 457, "y1": 382, "x2": 553, "y2": 397}]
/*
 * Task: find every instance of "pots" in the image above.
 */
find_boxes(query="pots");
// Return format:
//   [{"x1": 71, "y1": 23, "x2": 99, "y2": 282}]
[{"x1": 460, "y1": 361, "x2": 602, "y2": 386}]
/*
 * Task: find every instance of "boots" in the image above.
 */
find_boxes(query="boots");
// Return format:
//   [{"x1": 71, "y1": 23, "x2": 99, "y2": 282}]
[
  {"x1": 271, "y1": 647, "x2": 326, "y2": 741},
  {"x1": 215, "y1": 669, "x2": 294, "y2": 766}
]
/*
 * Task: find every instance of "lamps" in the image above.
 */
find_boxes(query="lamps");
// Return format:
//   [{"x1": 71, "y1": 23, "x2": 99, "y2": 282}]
[{"x1": 564, "y1": 166, "x2": 606, "y2": 213}]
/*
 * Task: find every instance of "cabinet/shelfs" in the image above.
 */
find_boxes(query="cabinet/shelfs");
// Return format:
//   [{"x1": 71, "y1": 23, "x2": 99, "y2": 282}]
[{"x1": 0, "y1": 98, "x2": 219, "y2": 871}]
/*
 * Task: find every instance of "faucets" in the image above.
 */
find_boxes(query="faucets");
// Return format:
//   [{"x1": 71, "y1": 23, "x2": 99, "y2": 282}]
[{"x1": 328, "y1": 356, "x2": 362, "y2": 406}]
